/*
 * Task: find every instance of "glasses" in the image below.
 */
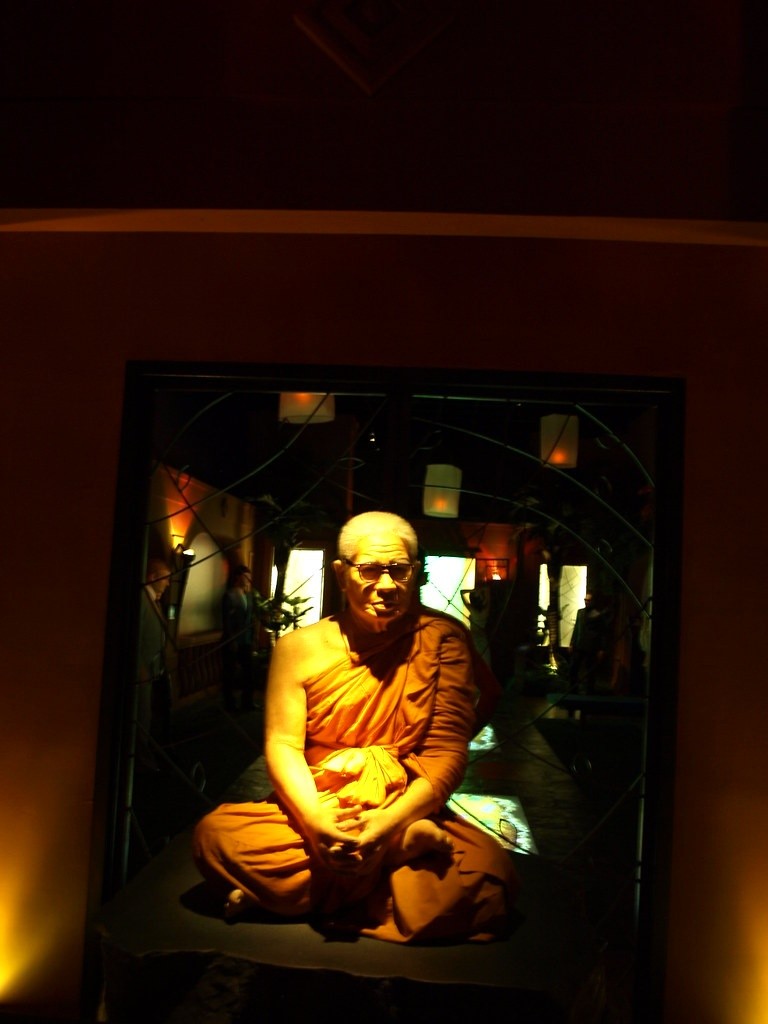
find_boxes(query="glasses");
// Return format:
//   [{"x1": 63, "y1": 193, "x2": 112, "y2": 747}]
[{"x1": 341, "y1": 556, "x2": 418, "y2": 584}]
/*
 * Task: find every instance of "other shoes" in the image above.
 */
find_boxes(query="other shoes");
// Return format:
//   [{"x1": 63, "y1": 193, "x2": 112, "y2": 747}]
[
  {"x1": 223, "y1": 704, "x2": 241, "y2": 716},
  {"x1": 586, "y1": 687, "x2": 599, "y2": 695},
  {"x1": 241, "y1": 702, "x2": 262, "y2": 712},
  {"x1": 570, "y1": 687, "x2": 579, "y2": 693},
  {"x1": 157, "y1": 738, "x2": 176, "y2": 747}
]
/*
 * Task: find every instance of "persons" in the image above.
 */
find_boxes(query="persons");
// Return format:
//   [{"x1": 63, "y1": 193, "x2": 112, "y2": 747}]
[
  {"x1": 194, "y1": 509, "x2": 520, "y2": 938},
  {"x1": 140, "y1": 559, "x2": 174, "y2": 770},
  {"x1": 461, "y1": 588, "x2": 490, "y2": 664},
  {"x1": 568, "y1": 590, "x2": 607, "y2": 688},
  {"x1": 225, "y1": 567, "x2": 266, "y2": 712}
]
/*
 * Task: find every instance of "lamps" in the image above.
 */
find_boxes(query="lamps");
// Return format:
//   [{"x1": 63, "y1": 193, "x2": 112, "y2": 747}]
[
  {"x1": 539, "y1": 414, "x2": 577, "y2": 467},
  {"x1": 422, "y1": 465, "x2": 461, "y2": 518},
  {"x1": 182, "y1": 549, "x2": 196, "y2": 562},
  {"x1": 278, "y1": 393, "x2": 335, "y2": 423}
]
